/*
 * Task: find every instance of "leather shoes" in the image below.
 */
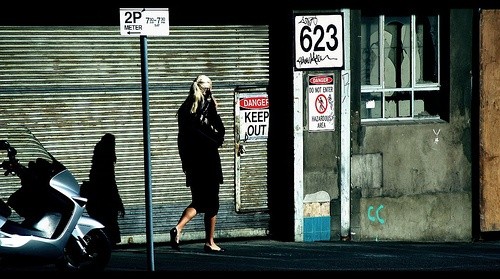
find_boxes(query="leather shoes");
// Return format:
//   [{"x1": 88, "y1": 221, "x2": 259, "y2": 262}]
[
  {"x1": 169, "y1": 227, "x2": 182, "y2": 250},
  {"x1": 204, "y1": 243, "x2": 228, "y2": 254}
]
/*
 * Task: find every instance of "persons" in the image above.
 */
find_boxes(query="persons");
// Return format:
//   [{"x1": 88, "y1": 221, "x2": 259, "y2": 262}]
[{"x1": 170, "y1": 75, "x2": 226, "y2": 253}]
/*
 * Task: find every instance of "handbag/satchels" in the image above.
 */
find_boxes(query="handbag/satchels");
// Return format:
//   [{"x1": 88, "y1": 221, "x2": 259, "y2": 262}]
[{"x1": 195, "y1": 103, "x2": 224, "y2": 148}]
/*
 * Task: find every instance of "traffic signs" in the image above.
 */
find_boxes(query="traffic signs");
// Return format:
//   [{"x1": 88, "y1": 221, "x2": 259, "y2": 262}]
[{"x1": 119, "y1": 8, "x2": 170, "y2": 37}]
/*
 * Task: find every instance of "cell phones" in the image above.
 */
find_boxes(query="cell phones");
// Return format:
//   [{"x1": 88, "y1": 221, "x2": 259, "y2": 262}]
[{"x1": 204, "y1": 89, "x2": 213, "y2": 99}]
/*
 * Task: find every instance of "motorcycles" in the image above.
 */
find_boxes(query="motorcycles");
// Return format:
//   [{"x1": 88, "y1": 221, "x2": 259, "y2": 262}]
[{"x1": 0, "y1": 123, "x2": 118, "y2": 277}]
